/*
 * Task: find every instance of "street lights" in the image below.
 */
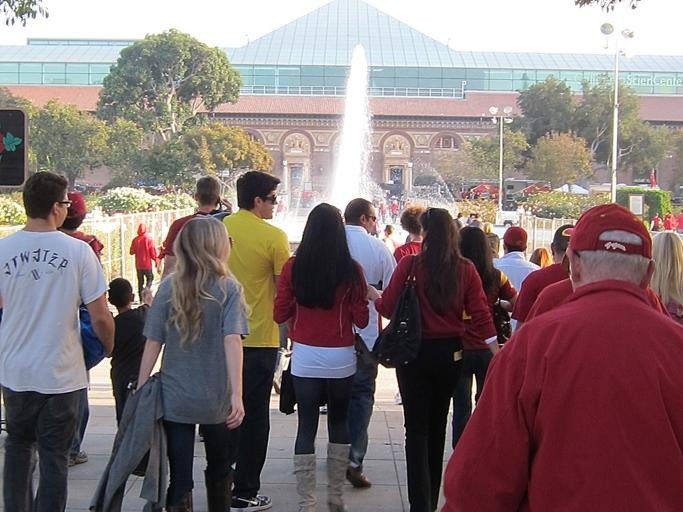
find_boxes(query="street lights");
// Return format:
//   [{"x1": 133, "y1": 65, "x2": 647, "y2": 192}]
[
  {"x1": 598, "y1": 18, "x2": 635, "y2": 202},
  {"x1": 488, "y1": 103, "x2": 513, "y2": 212}
]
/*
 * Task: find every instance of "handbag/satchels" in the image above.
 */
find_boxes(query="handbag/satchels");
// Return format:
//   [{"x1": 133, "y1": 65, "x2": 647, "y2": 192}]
[
  {"x1": 492, "y1": 298, "x2": 514, "y2": 348},
  {"x1": 370, "y1": 254, "x2": 418, "y2": 368}
]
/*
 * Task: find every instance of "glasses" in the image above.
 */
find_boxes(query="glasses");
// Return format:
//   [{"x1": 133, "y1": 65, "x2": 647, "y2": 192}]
[
  {"x1": 260, "y1": 193, "x2": 278, "y2": 206},
  {"x1": 367, "y1": 215, "x2": 379, "y2": 222}
]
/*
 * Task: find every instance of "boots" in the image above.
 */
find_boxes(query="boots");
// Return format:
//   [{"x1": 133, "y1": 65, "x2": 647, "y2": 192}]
[
  {"x1": 292, "y1": 452, "x2": 315, "y2": 508},
  {"x1": 206, "y1": 470, "x2": 230, "y2": 512},
  {"x1": 165, "y1": 489, "x2": 191, "y2": 512},
  {"x1": 325, "y1": 442, "x2": 351, "y2": 512}
]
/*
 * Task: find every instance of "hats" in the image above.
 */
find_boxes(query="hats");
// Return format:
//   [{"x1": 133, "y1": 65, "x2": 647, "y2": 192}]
[
  {"x1": 502, "y1": 225, "x2": 528, "y2": 253},
  {"x1": 64, "y1": 192, "x2": 86, "y2": 215},
  {"x1": 554, "y1": 223, "x2": 575, "y2": 252},
  {"x1": 567, "y1": 201, "x2": 655, "y2": 263}
]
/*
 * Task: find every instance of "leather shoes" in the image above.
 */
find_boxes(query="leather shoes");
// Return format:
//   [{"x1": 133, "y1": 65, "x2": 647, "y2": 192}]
[{"x1": 346, "y1": 468, "x2": 372, "y2": 494}]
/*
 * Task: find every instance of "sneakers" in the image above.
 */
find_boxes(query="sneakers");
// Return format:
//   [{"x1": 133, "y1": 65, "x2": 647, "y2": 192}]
[
  {"x1": 230, "y1": 492, "x2": 275, "y2": 512},
  {"x1": 68, "y1": 453, "x2": 88, "y2": 465},
  {"x1": 394, "y1": 389, "x2": 402, "y2": 403}
]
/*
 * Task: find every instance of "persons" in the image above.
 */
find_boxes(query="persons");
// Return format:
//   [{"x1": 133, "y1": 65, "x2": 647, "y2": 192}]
[
  {"x1": 107, "y1": 277, "x2": 155, "y2": 430},
  {"x1": 513, "y1": 223, "x2": 581, "y2": 341},
  {"x1": 337, "y1": 198, "x2": 400, "y2": 488},
  {"x1": 380, "y1": 223, "x2": 399, "y2": 253},
  {"x1": 510, "y1": 224, "x2": 574, "y2": 329},
  {"x1": 492, "y1": 227, "x2": 542, "y2": 334},
  {"x1": 648, "y1": 209, "x2": 682, "y2": 232},
  {"x1": 130, "y1": 223, "x2": 160, "y2": 304},
  {"x1": 159, "y1": 177, "x2": 232, "y2": 261},
  {"x1": 133, "y1": 216, "x2": 250, "y2": 512},
  {"x1": 523, "y1": 278, "x2": 573, "y2": 322},
  {"x1": 221, "y1": 170, "x2": 290, "y2": 511},
  {"x1": 57, "y1": 192, "x2": 106, "y2": 468},
  {"x1": 490, "y1": 224, "x2": 548, "y2": 335},
  {"x1": 650, "y1": 228, "x2": 683, "y2": 320},
  {"x1": 0, "y1": 171, "x2": 116, "y2": 512},
  {"x1": 438, "y1": 202, "x2": 683, "y2": 510},
  {"x1": 365, "y1": 207, "x2": 500, "y2": 512},
  {"x1": 393, "y1": 206, "x2": 425, "y2": 263},
  {"x1": 273, "y1": 202, "x2": 368, "y2": 512},
  {"x1": 529, "y1": 248, "x2": 550, "y2": 268},
  {"x1": 452, "y1": 226, "x2": 518, "y2": 447},
  {"x1": 378, "y1": 200, "x2": 400, "y2": 223}
]
